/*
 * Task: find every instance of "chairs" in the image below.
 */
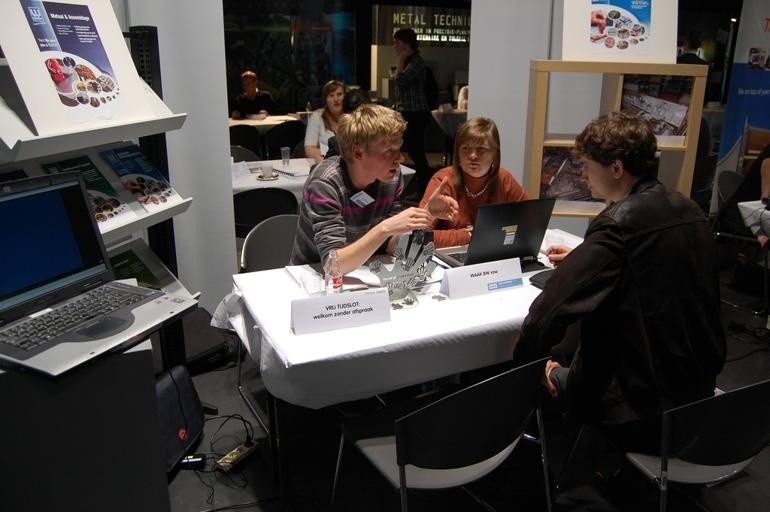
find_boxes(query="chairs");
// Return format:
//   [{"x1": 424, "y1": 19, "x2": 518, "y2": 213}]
[
  {"x1": 229, "y1": 120, "x2": 307, "y2": 162},
  {"x1": 237, "y1": 213, "x2": 301, "y2": 438},
  {"x1": 692, "y1": 115, "x2": 710, "y2": 193},
  {"x1": 329, "y1": 354, "x2": 555, "y2": 512},
  {"x1": 297, "y1": 85, "x2": 322, "y2": 110},
  {"x1": 713, "y1": 170, "x2": 769, "y2": 315},
  {"x1": 623, "y1": 378, "x2": 770, "y2": 512},
  {"x1": 233, "y1": 187, "x2": 299, "y2": 239}
]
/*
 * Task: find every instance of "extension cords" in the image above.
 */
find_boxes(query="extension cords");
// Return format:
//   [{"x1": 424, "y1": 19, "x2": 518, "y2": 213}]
[{"x1": 215, "y1": 440, "x2": 260, "y2": 474}]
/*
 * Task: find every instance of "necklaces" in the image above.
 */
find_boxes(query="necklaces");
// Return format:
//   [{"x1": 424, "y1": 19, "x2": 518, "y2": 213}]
[{"x1": 462, "y1": 178, "x2": 492, "y2": 198}]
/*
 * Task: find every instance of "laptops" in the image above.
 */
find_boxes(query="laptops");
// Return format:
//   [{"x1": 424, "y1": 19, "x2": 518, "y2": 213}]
[
  {"x1": 435, "y1": 198, "x2": 555, "y2": 268},
  {"x1": 0, "y1": 172, "x2": 198, "y2": 376}
]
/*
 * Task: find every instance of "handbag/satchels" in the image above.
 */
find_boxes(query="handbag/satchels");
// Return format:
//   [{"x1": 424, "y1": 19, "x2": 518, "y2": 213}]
[
  {"x1": 424, "y1": 66, "x2": 438, "y2": 109},
  {"x1": 183, "y1": 308, "x2": 247, "y2": 376},
  {"x1": 156, "y1": 366, "x2": 205, "y2": 479}
]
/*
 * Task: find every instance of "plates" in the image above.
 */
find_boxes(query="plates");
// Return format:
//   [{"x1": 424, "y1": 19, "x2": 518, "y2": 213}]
[{"x1": 255, "y1": 173, "x2": 279, "y2": 181}]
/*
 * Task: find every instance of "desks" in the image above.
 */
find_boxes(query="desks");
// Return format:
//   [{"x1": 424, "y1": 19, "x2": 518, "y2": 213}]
[
  {"x1": 431, "y1": 108, "x2": 467, "y2": 166},
  {"x1": 229, "y1": 114, "x2": 297, "y2": 127},
  {"x1": 230, "y1": 228, "x2": 584, "y2": 497},
  {"x1": 231, "y1": 158, "x2": 416, "y2": 207}
]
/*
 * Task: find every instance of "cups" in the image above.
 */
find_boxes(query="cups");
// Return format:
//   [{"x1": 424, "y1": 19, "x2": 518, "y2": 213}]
[
  {"x1": 260, "y1": 163, "x2": 273, "y2": 178},
  {"x1": 281, "y1": 146, "x2": 291, "y2": 167}
]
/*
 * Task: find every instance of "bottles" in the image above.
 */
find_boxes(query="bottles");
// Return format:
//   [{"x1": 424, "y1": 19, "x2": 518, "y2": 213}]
[
  {"x1": 305, "y1": 101, "x2": 312, "y2": 112},
  {"x1": 324, "y1": 248, "x2": 344, "y2": 297}
]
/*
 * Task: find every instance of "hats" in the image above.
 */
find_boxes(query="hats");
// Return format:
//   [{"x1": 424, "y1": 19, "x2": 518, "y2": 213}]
[{"x1": 394, "y1": 29, "x2": 415, "y2": 42}]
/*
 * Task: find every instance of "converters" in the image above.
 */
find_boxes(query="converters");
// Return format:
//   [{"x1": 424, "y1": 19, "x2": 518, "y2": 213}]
[{"x1": 179, "y1": 454, "x2": 206, "y2": 467}]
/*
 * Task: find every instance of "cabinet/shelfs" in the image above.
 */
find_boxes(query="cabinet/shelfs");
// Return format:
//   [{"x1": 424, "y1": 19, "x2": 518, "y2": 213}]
[
  {"x1": 523, "y1": 60, "x2": 710, "y2": 217},
  {"x1": 0, "y1": 25, "x2": 219, "y2": 416}
]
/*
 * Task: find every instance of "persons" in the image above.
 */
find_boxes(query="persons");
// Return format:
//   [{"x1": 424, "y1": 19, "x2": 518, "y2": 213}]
[
  {"x1": 287, "y1": 102, "x2": 434, "y2": 282},
  {"x1": 230, "y1": 68, "x2": 280, "y2": 154},
  {"x1": 513, "y1": 111, "x2": 725, "y2": 497},
  {"x1": 305, "y1": 77, "x2": 346, "y2": 161},
  {"x1": 675, "y1": 26, "x2": 710, "y2": 95},
  {"x1": 385, "y1": 27, "x2": 450, "y2": 182},
  {"x1": 417, "y1": 118, "x2": 527, "y2": 246},
  {"x1": 308, "y1": 89, "x2": 372, "y2": 166}
]
